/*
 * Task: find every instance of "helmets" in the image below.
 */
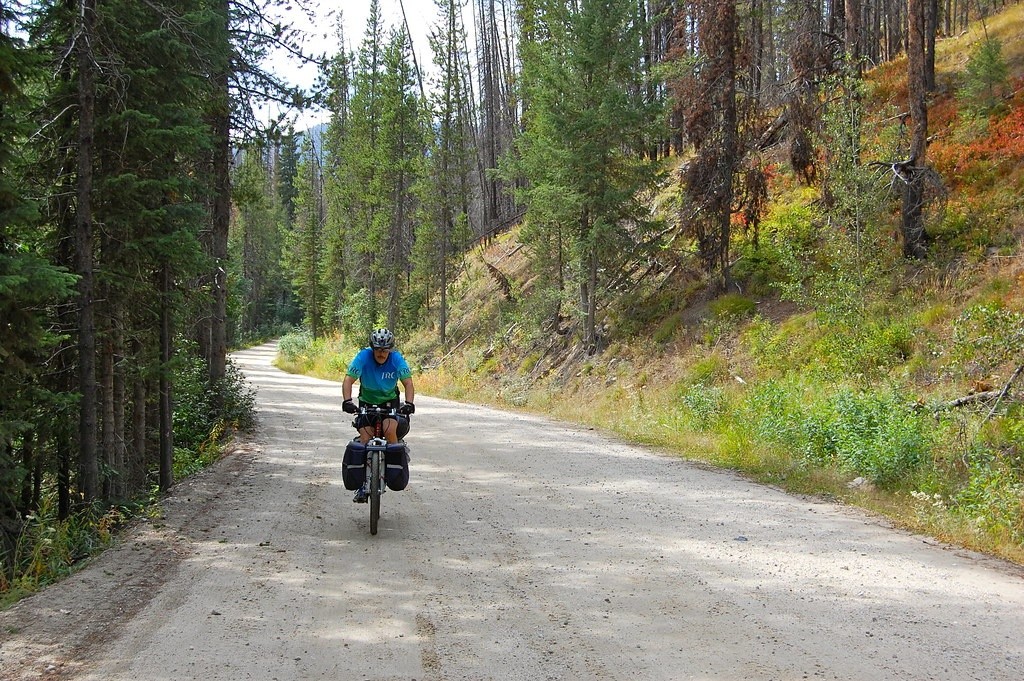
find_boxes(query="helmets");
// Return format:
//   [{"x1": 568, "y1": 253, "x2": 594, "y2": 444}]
[{"x1": 369, "y1": 328, "x2": 395, "y2": 348}]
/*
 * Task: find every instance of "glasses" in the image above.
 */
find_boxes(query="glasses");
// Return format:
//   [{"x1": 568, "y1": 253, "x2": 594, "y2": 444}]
[{"x1": 371, "y1": 346, "x2": 391, "y2": 353}]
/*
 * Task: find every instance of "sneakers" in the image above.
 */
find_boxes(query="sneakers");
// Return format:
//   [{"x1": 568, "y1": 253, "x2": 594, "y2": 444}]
[{"x1": 353, "y1": 487, "x2": 368, "y2": 503}]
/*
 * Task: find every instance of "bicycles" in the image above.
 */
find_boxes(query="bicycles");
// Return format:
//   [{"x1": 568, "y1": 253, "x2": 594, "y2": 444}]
[{"x1": 352, "y1": 407, "x2": 408, "y2": 535}]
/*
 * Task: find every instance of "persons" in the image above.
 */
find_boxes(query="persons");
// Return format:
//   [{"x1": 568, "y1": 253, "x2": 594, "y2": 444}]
[{"x1": 343, "y1": 329, "x2": 415, "y2": 503}]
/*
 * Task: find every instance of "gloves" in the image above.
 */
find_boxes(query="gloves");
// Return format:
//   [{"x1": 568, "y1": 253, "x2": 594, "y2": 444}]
[
  {"x1": 400, "y1": 401, "x2": 415, "y2": 414},
  {"x1": 342, "y1": 399, "x2": 356, "y2": 413}
]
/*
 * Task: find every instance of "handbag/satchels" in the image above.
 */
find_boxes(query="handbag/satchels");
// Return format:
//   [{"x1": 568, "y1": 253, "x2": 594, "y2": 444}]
[{"x1": 341, "y1": 440, "x2": 409, "y2": 491}]
